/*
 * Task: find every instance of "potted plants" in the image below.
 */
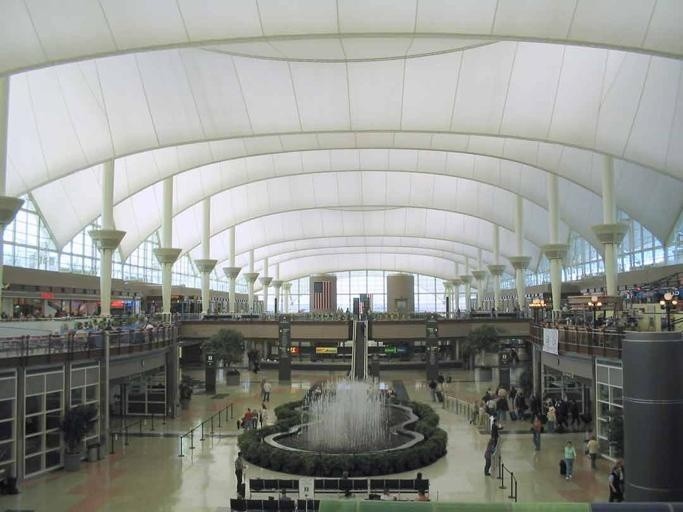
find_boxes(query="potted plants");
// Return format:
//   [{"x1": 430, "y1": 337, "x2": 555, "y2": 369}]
[
  {"x1": 178, "y1": 384, "x2": 193, "y2": 409},
  {"x1": 225, "y1": 368, "x2": 239, "y2": 386},
  {"x1": 56, "y1": 405, "x2": 91, "y2": 472}
]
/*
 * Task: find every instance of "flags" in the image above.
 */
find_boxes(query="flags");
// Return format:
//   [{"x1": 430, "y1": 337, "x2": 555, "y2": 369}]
[
  {"x1": 358, "y1": 293, "x2": 372, "y2": 315},
  {"x1": 313, "y1": 281, "x2": 331, "y2": 312}
]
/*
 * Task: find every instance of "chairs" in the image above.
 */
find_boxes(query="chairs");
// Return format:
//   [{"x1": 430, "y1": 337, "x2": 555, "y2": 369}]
[
  {"x1": 229, "y1": 477, "x2": 431, "y2": 512},
  {"x1": 48, "y1": 321, "x2": 162, "y2": 352}
]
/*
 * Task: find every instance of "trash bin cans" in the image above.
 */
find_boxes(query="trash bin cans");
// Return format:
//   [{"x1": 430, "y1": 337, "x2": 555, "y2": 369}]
[
  {"x1": 87, "y1": 444, "x2": 99, "y2": 462},
  {"x1": 227, "y1": 371, "x2": 240, "y2": 385}
]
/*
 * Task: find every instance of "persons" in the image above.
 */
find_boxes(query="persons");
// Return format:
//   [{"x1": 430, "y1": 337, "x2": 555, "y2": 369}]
[
  {"x1": 0, "y1": 305, "x2": 67, "y2": 317},
  {"x1": 235, "y1": 378, "x2": 291, "y2": 501},
  {"x1": 78, "y1": 313, "x2": 154, "y2": 359},
  {"x1": 429, "y1": 305, "x2": 679, "y2": 503},
  {"x1": 336, "y1": 306, "x2": 351, "y2": 320},
  {"x1": 340, "y1": 471, "x2": 429, "y2": 501}
]
